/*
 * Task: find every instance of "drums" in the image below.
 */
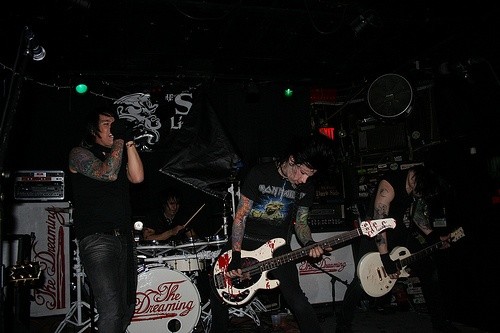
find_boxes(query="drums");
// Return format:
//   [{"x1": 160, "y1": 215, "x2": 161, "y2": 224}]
[
  {"x1": 123, "y1": 262, "x2": 202, "y2": 333},
  {"x1": 167, "y1": 253, "x2": 205, "y2": 275},
  {"x1": 134, "y1": 234, "x2": 228, "y2": 253}
]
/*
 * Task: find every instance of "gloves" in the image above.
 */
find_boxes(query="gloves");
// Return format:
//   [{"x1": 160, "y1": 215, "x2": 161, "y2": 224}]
[{"x1": 110, "y1": 119, "x2": 135, "y2": 144}]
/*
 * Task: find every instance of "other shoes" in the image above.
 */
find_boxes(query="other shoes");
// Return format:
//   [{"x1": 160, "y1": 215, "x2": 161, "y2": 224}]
[
  {"x1": 431, "y1": 315, "x2": 461, "y2": 333},
  {"x1": 336, "y1": 306, "x2": 354, "y2": 333}
]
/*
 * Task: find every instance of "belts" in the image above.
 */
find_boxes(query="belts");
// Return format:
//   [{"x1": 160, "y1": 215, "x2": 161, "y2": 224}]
[{"x1": 99, "y1": 229, "x2": 133, "y2": 237}]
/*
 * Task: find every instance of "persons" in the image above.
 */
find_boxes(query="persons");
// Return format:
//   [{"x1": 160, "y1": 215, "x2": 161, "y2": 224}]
[
  {"x1": 142, "y1": 186, "x2": 200, "y2": 243},
  {"x1": 210, "y1": 133, "x2": 333, "y2": 331},
  {"x1": 63, "y1": 104, "x2": 145, "y2": 332},
  {"x1": 339, "y1": 160, "x2": 454, "y2": 332}
]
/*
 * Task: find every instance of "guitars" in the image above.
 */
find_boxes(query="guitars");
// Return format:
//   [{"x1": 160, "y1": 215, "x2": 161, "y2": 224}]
[
  {"x1": 211, "y1": 212, "x2": 397, "y2": 309},
  {"x1": 356, "y1": 225, "x2": 466, "y2": 299}
]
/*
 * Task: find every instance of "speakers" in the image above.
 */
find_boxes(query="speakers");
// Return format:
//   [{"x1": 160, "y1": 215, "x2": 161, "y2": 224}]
[{"x1": 0, "y1": 232, "x2": 35, "y2": 333}]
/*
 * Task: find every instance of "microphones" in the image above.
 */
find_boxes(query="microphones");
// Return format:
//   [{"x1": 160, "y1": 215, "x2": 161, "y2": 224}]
[
  {"x1": 131, "y1": 123, "x2": 144, "y2": 134},
  {"x1": 24, "y1": 28, "x2": 47, "y2": 62}
]
